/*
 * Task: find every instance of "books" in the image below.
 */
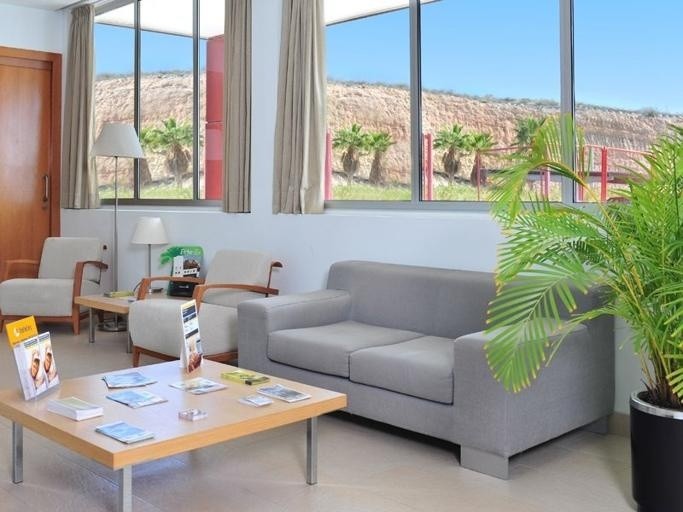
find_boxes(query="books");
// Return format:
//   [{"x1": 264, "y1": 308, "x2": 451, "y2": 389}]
[
  {"x1": 259, "y1": 384, "x2": 312, "y2": 402},
  {"x1": 169, "y1": 378, "x2": 227, "y2": 394},
  {"x1": 48, "y1": 396, "x2": 103, "y2": 421},
  {"x1": 106, "y1": 389, "x2": 168, "y2": 407},
  {"x1": 16, "y1": 332, "x2": 60, "y2": 399},
  {"x1": 221, "y1": 370, "x2": 272, "y2": 385},
  {"x1": 97, "y1": 420, "x2": 154, "y2": 444},
  {"x1": 104, "y1": 371, "x2": 158, "y2": 387}
]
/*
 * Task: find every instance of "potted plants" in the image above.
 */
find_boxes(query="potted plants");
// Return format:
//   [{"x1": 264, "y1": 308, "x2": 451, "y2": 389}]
[{"x1": 484, "y1": 113, "x2": 682, "y2": 511}]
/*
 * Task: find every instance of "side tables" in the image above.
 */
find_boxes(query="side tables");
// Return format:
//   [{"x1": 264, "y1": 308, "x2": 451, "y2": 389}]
[{"x1": 73, "y1": 290, "x2": 168, "y2": 353}]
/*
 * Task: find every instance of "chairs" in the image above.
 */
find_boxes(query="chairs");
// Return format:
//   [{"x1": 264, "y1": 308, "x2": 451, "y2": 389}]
[
  {"x1": 0, "y1": 236, "x2": 112, "y2": 333},
  {"x1": 128, "y1": 246, "x2": 284, "y2": 367}
]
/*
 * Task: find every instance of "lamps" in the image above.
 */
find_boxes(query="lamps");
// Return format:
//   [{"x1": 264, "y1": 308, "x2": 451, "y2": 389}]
[
  {"x1": 89, "y1": 121, "x2": 146, "y2": 331},
  {"x1": 131, "y1": 217, "x2": 170, "y2": 292}
]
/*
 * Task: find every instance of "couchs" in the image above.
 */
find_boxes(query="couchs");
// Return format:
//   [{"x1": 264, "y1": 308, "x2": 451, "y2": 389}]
[{"x1": 236, "y1": 260, "x2": 615, "y2": 479}]
